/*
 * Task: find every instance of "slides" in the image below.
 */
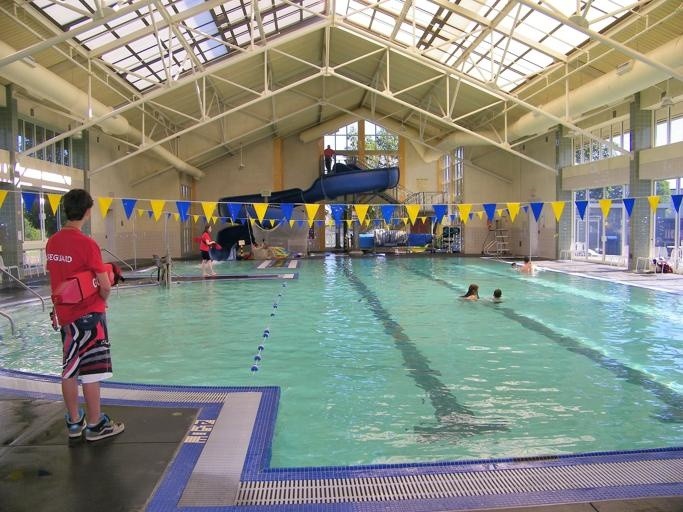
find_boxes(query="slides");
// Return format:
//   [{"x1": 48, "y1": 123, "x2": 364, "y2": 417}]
[{"x1": 208, "y1": 163, "x2": 400, "y2": 261}]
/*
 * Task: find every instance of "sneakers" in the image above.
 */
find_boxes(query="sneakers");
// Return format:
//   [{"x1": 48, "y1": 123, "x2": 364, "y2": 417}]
[
  {"x1": 64, "y1": 408, "x2": 86, "y2": 438},
  {"x1": 85, "y1": 413, "x2": 125, "y2": 441}
]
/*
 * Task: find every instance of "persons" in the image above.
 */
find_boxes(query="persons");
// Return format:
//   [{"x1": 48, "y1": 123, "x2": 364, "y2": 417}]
[
  {"x1": 512, "y1": 263, "x2": 517, "y2": 270},
  {"x1": 324, "y1": 145, "x2": 335, "y2": 173},
  {"x1": 519, "y1": 256, "x2": 532, "y2": 274},
  {"x1": 460, "y1": 285, "x2": 478, "y2": 300},
  {"x1": 492, "y1": 289, "x2": 502, "y2": 300},
  {"x1": 46, "y1": 189, "x2": 125, "y2": 441},
  {"x1": 199, "y1": 225, "x2": 216, "y2": 276}
]
